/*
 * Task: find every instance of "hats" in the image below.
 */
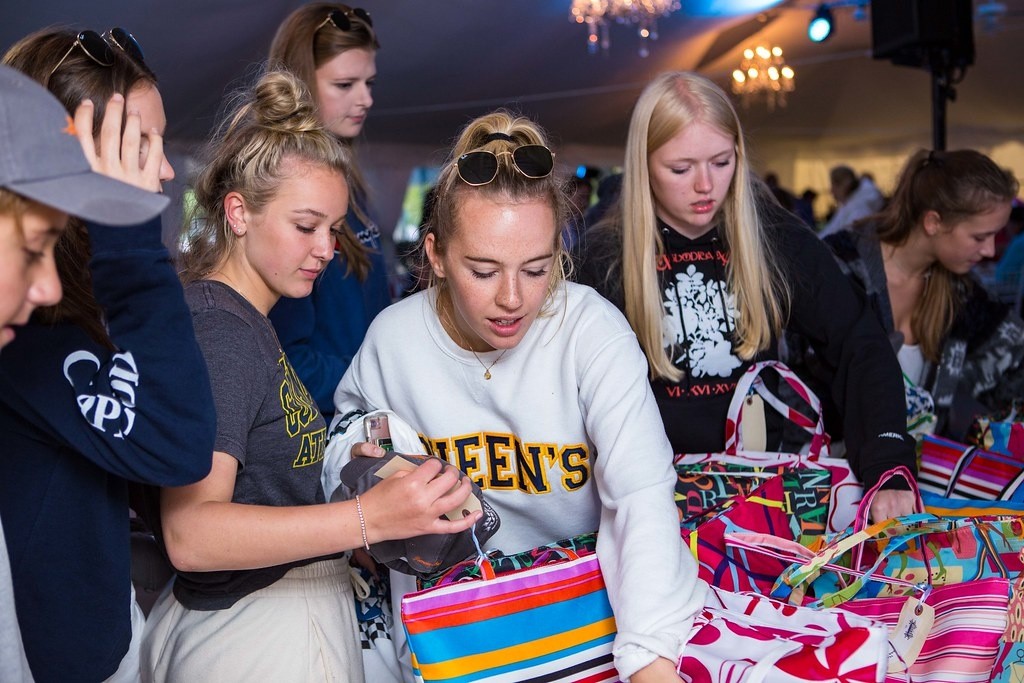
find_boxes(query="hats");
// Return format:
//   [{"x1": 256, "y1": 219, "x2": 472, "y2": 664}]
[{"x1": 0, "y1": 65, "x2": 170, "y2": 227}]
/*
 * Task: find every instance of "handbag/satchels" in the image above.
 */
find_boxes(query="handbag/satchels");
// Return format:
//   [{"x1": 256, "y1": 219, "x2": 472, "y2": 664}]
[
  {"x1": 400, "y1": 361, "x2": 1024, "y2": 683},
  {"x1": 330, "y1": 453, "x2": 501, "y2": 582}
]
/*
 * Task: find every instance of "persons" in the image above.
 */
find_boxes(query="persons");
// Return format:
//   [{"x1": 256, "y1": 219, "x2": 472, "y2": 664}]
[
  {"x1": 0, "y1": 22, "x2": 217, "y2": 683},
  {"x1": 137, "y1": 64, "x2": 484, "y2": 683},
  {"x1": 320, "y1": 106, "x2": 709, "y2": 682},
  {"x1": 395, "y1": 162, "x2": 1024, "y2": 334},
  {"x1": 576, "y1": 71, "x2": 926, "y2": 526},
  {"x1": 267, "y1": 0, "x2": 390, "y2": 429},
  {"x1": 0, "y1": 63, "x2": 174, "y2": 683},
  {"x1": 819, "y1": 148, "x2": 1024, "y2": 444}
]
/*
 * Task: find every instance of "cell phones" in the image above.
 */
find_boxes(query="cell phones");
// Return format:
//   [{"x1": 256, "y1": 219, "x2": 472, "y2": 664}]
[{"x1": 363, "y1": 413, "x2": 395, "y2": 453}]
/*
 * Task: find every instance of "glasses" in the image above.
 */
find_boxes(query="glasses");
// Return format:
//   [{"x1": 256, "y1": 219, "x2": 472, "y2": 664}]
[
  {"x1": 314, "y1": 8, "x2": 373, "y2": 33},
  {"x1": 436, "y1": 144, "x2": 556, "y2": 199},
  {"x1": 49, "y1": 27, "x2": 145, "y2": 77}
]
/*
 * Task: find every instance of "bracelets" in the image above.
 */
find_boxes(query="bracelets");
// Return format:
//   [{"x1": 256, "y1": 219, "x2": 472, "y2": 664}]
[{"x1": 356, "y1": 495, "x2": 369, "y2": 551}]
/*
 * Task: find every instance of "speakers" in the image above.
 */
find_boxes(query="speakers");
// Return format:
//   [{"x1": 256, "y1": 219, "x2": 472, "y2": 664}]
[{"x1": 869, "y1": 0, "x2": 975, "y2": 66}]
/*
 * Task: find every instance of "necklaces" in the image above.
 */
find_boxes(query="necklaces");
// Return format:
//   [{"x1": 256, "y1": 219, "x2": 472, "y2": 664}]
[
  {"x1": 208, "y1": 268, "x2": 283, "y2": 354},
  {"x1": 887, "y1": 246, "x2": 931, "y2": 280},
  {"x1": 459, "y1": 327, "x2": 505, "y2": 378}
]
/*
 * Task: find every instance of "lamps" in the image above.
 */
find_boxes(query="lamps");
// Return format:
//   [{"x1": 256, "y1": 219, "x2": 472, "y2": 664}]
[
  {"x1": 807, "y1": 0, "x2": 836, "y2": 46},
  {"x1": 730, "y1": 44, "x2": 796, "y2": 109},
  {"x1": 567, "y1": 0, "x2": 682, "y2": 58}
]
